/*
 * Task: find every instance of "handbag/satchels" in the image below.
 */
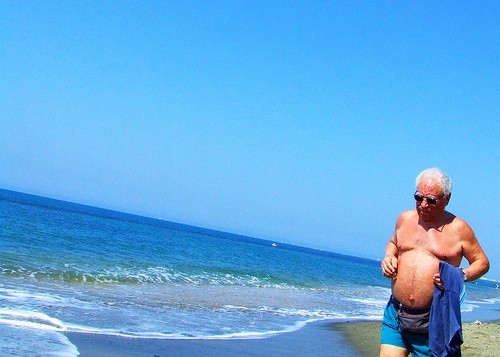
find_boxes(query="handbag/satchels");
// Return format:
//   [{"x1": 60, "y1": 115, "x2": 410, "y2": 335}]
[{"x1": 395, "y1": 309, "x2": 430, "y2": 336}]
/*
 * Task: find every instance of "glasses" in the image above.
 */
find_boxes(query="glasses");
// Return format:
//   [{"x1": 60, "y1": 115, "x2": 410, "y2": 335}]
[{"x1": 414, "y1": 190, "x2": 445, "y2": 205}]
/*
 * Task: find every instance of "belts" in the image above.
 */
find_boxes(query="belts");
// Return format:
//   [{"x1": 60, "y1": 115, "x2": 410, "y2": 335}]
[{"x1": 390, "y1": 295, "x2": 431, "y2": 315}]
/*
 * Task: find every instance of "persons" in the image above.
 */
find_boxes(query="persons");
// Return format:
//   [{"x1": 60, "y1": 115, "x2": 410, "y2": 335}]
[{"x1": 379, "y1": 166, "x2": 490, "y2": 357}]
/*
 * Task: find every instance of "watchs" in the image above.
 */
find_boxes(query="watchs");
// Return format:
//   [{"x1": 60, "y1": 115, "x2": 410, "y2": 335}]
[{"x1": 462, "y1": 270, "x2": 467, "y2": 282}]
